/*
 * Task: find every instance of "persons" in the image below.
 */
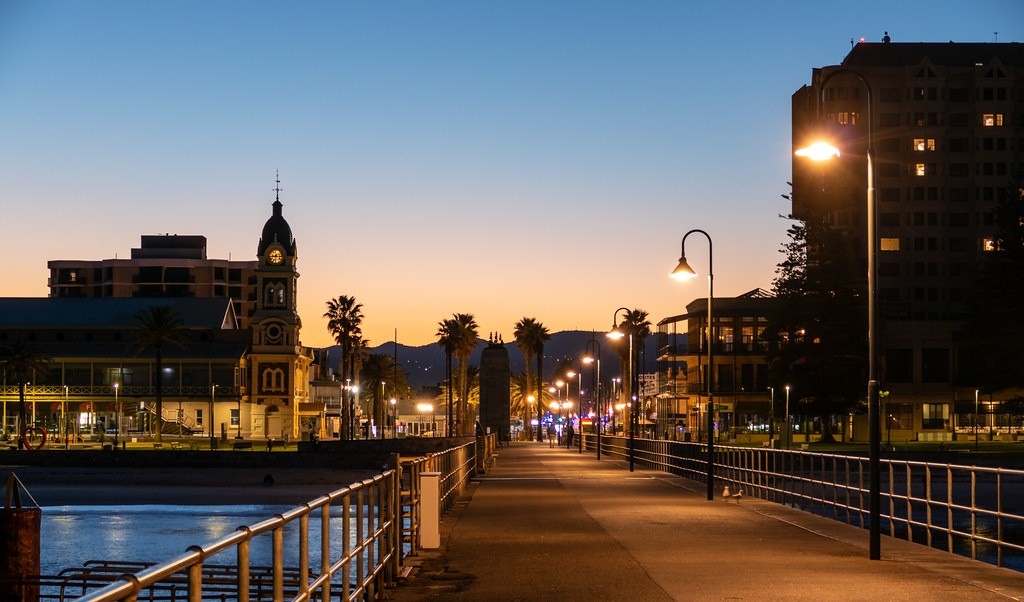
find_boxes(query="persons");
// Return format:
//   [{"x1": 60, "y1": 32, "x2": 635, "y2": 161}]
[
  {"x1": 547, "y1": 424, "x2": 557, "y2": 448},
  {"x1": 566, "y1": 425, "x2": 574, "y2": 445}
]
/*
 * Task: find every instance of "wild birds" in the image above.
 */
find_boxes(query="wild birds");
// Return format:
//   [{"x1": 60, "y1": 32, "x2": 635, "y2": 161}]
[{"x1": 723, "y1": 486, "x2": 744, "y2": 504}]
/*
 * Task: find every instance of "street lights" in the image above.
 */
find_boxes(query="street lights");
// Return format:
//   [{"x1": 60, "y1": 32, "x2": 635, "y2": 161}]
[
  {"x1": 605, "y1": 308, "x2": 635, "y2": 471},
  {"x1": 566, "y1": 358, "x2": 582, "y2": 454},
  {"x1": 670, "y1": 230, "x2": 715, "y2": 501},
  {"x1": 793, "y1": 140, "x2": 882, "y2": 561},
  {"x1": 550, "y1": 379, "x2": 571, "y2": 450},
  {"x1": 583, "y1": 339, "x2": 600, "y2": 461}
]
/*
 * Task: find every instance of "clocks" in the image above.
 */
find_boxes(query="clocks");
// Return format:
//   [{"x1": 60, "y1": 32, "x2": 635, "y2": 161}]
[{"x1": 267, "y1": 247, "x2": 285, "y2": 265}]
